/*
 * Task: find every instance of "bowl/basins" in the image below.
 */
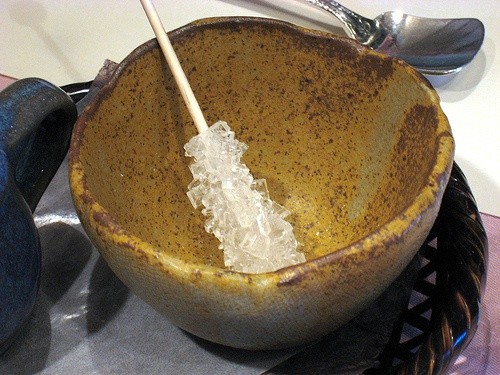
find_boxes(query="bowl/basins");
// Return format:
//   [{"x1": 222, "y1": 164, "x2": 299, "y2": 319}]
[{"x1": 69, "y1": 17, "x2": 456, "y2": 350}]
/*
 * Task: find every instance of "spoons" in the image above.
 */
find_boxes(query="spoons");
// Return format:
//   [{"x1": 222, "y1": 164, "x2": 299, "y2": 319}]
[{"x1": 301, "y1": 0, "x2": 485, "y2": 75}]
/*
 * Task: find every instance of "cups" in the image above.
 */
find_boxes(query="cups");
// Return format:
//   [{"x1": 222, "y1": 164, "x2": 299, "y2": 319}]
[{"x1": 0, "y1": 78, "x2": 75, "y2": 357}]
